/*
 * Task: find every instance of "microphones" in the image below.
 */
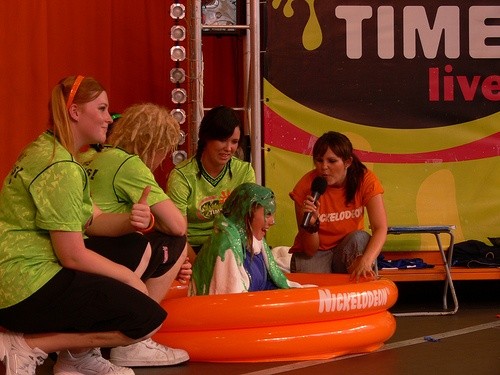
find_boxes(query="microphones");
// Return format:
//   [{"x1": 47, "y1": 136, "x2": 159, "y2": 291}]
[{"x1": 300, "y1": 176, "x2": 328, "y2": 230}]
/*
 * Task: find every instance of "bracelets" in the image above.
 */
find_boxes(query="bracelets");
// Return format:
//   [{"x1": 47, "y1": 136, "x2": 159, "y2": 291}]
[
  {"x1": 302, "y1": 218, "x2": 322, "y2": 234},
  {"x1": 134, "y1": 213, "x2": 156, "y2": 232}
]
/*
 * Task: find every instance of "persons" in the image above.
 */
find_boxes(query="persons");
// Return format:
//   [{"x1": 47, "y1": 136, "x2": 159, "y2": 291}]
[
  {"x1": 84, "y1": 103, "x2": 189, "y2": 366},
  {"x1": 166, "y1": 105, "x2": 256, "y2": 256},
  {"x1": 1, "y1": 75, "x2": 168, "y2": 374},
  {"x1": 79, "y1": 112, "x2": 124, "y2": 167},
  {"x1": 187, "y1": 182, "x2": 276, "y2": 296},
  {"x1": 289, "y1": 130, "x2": 388, "y2": 284}
]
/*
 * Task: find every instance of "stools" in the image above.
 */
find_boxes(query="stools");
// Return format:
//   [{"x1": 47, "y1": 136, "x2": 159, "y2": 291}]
[{"x1": 368, "y1": 225, "x2": 458, "y2": 317}]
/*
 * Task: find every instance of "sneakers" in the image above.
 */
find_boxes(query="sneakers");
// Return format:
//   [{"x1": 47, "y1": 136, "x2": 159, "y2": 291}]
[
  {"x1": 53, "y1": 346, "x2": 135, "y2": 375},
  {"x1": 109, "y1": 338, "x2": 190, "y2": 366},
  {"x1": 0, "y1": 326, "x2": 48, "y2": 375}
]
